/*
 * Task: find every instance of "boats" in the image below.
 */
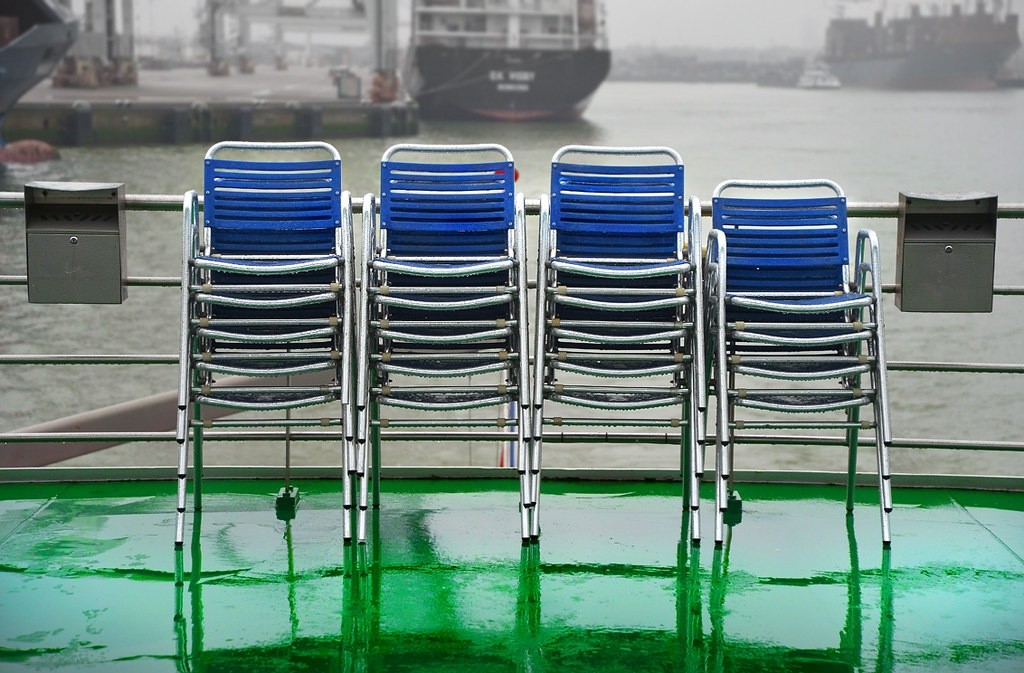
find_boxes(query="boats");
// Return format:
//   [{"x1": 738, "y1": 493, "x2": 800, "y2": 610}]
[
  {"x1": 400, "y1": 0, "x2": 612, "y2": 122},
  {"x1": 823, "y1": 0, "x2": 1021, "y2": 92},
  {"x1": 796, "y1": 70, "x2": 841, "y2": 90}
]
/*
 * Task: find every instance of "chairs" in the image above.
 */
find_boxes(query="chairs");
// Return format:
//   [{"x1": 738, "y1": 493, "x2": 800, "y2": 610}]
[{"x1": 171, "y1": 139, "x2": 897, "y2": 551}]
